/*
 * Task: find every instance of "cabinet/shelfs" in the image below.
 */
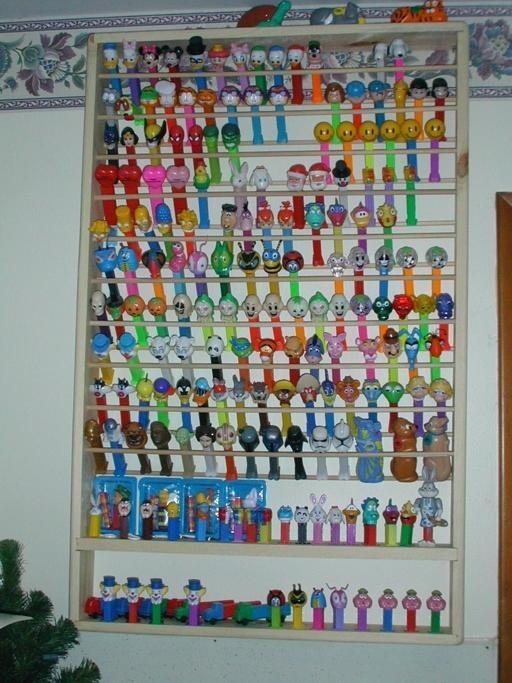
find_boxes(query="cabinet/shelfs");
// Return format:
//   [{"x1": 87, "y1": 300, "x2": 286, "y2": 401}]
[{"x1": 69, "y1": 21, "x2": 469, "y2": 647}]
